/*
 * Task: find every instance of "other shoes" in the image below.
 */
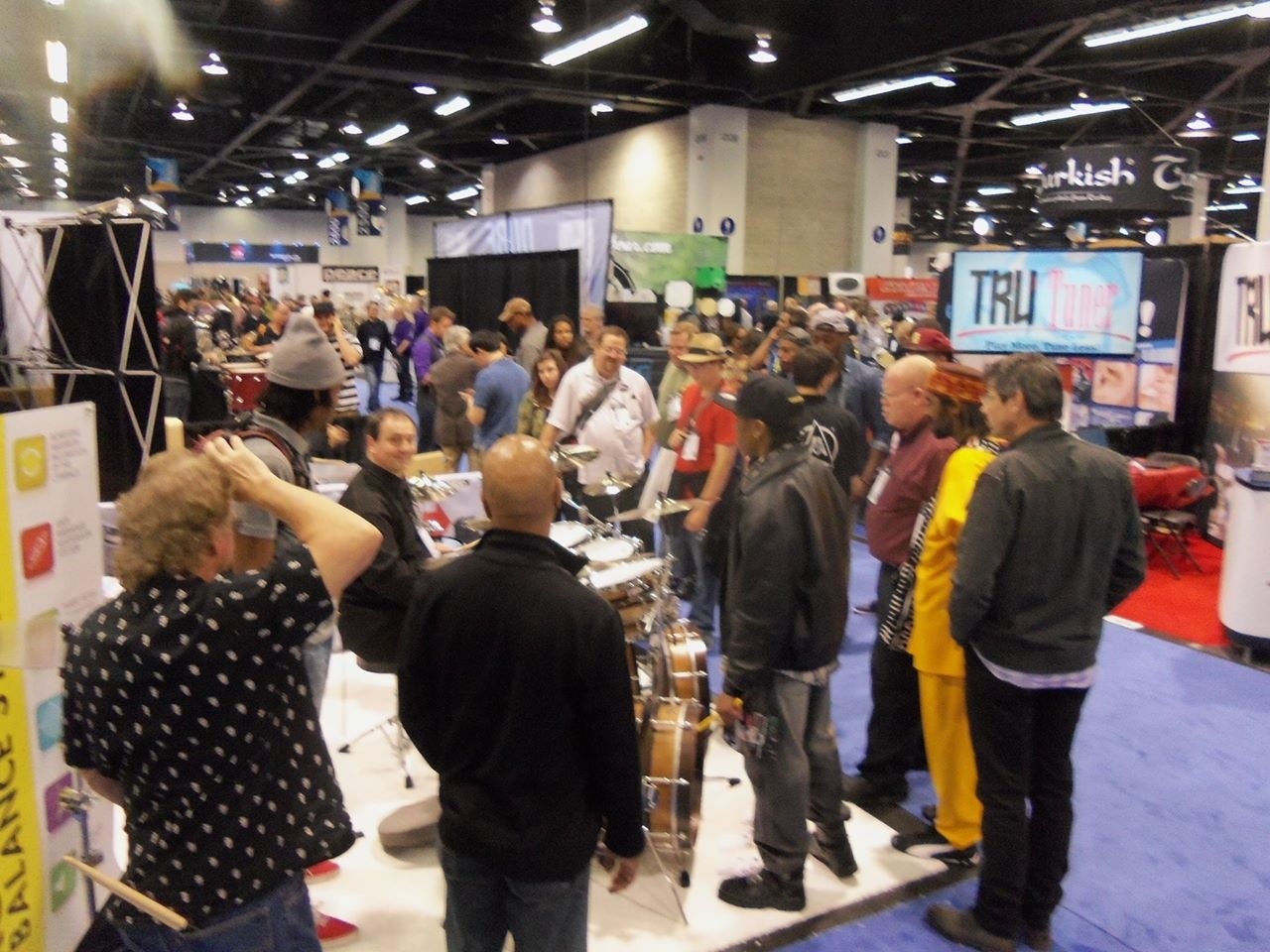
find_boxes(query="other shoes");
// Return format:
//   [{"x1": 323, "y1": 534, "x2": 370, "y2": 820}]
[
  {"x1": 667, "y1": 574, "x2": 714, "y2": 653},
  {"x1": 369, "y1": 394, "x2": 414, "y2": 414}
]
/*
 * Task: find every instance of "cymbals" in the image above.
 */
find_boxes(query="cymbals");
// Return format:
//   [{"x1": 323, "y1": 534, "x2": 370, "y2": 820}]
[
  {"x1": 582, "y1": 476, "x2": 635, "y2": 498},
  {"x1": 605, "y1": 497, "x2": 717, "y2": 523},
  {"x1": 547, "y1": 441, "x2": 601, "y2": 476}
]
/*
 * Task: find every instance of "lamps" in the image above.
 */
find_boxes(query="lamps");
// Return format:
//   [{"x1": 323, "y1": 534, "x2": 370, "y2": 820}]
[
  {"x1": 818, "y1": 61, "x2": 956, "y2": 106},
  {"x1": 1073, "y1": 0, "x2": 1258, "y2": 53},
  {"x1": 1, "y1": 0, "x2": 650, "y2": 211},
  {"x1": 930, "y1": 59, "x2": 1262, "y2": 244},
  {"x1": 749, "y1": 37, "x2": 779, "y2": 63}
]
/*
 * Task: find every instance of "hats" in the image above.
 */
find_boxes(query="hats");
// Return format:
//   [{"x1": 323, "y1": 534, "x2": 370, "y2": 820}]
[
  {"x1": 782, "y1": 326, "x2": 811, "y2": 346},
  {"x1": 264, "y1": 313, "x2": 347, "y2": 390},
  {"x1": 900, "y1": 328, "x2": 955, "y2": 354},
  {"x1": 923, "y1": 361, "x2": 990, "y2": 405},
  {"x1": 497, "y1": 297, "x2": 532, "y2": 322},
  {"x1": 676, "y1": 332, "x2": 729, "y2": 363},
  {"x1": 807, "y1": 310, "x2": 851, "y2": 333}
]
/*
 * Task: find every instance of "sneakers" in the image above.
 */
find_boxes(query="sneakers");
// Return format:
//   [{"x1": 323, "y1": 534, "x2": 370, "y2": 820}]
[
  {"x1": 804, "y1": 830, "x2": 858, "y2": 878},
  {"x1": 925, "y1": 902, "x2": 1054, "y2": 951},
  {"x1": 303, "y1": 859, "x2": 344, "y2": 883},
  {"x1": 891, "y1": 803, "x2": 980, "y2": 867},
  {"x1": 838, "y1": 767, "x2": 908, "y2": 807},
  {"x1": 853, "y1": 599, "x2": 883, "y2": 617},
  {"x1": 716, "y1": 869, "x2": 806, "y2": 913},
  {"x1": 308, "y1": 904, "x2": 362, "y2": 951}
]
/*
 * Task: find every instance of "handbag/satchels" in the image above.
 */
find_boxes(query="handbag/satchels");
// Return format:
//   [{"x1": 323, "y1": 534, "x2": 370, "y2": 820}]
[{"x1": 556, "y1": 436, "x2": 579, "y2": 478}]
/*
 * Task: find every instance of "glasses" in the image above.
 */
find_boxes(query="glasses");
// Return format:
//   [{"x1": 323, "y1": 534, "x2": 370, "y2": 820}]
[
  {"x1": 663, "y1": 343, "x2": 688, "y2": 351},
  {"x1": 602, "y1": 347, "x2": 628, "y2": 356},
  {"x1": 686, "y1": 361, "x2": 716, "y2": 369},
  {"x1": 876, "y1": 389, "x2": 912, "y2": 403}
]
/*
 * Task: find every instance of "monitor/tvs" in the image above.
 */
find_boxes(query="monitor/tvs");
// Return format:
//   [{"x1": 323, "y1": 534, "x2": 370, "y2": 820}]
[{"x1": 947, "y1": 250, "x2": 1143, "y2": 355}]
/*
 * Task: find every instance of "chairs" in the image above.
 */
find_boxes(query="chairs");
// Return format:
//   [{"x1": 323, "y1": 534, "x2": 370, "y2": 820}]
[{"x1": 1125, "y1": 450, "x2": 1216, "y2": 581}]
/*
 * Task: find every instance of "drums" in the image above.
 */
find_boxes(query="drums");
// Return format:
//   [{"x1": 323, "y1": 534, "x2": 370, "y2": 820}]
[
  {"x1": 631, "y1": 696, "x2": 710, "y2": 883},
  {"x1": 585, "y1": 555, "x2": 676, "y2": 607},
  {"x1": 548, "y1": 522, "x2": 592, "y2": 550},
  {"x1": 573, "y1": 534, "x2": 642, "y2": 568},
  {"x1": 652, "y1": 616, "x2": 712, "y2": 712}
]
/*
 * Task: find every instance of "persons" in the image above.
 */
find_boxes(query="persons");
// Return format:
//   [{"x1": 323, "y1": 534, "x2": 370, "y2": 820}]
[
  {"x1": 889, "y1": 362, "x2": 999, "y2": 863},
  {"x1": 216, "y1": 308, "x2": 360, "y2": 940},
  {"x1": 842, "y1": 353, "x2": 957, "y2": 804},
  {"x1": 160, "y1": 279, "x2": 950, "y2": 661},
  {"x1": 700, "y1": 374, "x2": 857, "y2": 911},
  {"x1": 397, "y1": 434, "x2": 647, "y2": 952},
  {"x1": 61, "y1": 437, "x2": 382, "y2": 952},
  {"x1": 925, "y1": 353, "x2": 1142, "y2": 952}
]
